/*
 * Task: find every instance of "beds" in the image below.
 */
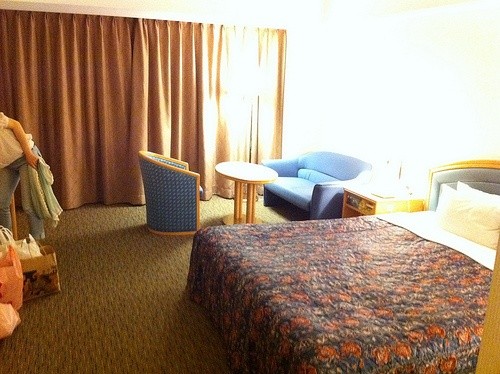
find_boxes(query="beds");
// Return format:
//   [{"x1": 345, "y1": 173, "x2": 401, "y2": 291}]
[{"x1": 187, "y1": 160, "x2": 500, "y2": 374}]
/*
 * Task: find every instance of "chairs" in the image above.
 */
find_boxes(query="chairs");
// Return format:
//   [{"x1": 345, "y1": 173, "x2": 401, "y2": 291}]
[{"x1": 138, "y1": 152, "x2": 203, "y2": 236}]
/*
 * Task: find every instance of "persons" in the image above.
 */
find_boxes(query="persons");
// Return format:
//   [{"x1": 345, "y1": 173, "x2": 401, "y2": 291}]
[{"x1": 0, "y1": 112, "x2": 64, "y2": 247}]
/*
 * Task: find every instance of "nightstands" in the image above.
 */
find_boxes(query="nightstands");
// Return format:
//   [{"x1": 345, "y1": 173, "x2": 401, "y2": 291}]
[{"x1": 343, "y1": 186, "x2": 425, "y2": 219}]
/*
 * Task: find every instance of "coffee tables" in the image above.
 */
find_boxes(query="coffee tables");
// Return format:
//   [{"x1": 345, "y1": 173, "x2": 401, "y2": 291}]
[{"x1": 215, "y1": 161, "x2": 277, "y2": 226}]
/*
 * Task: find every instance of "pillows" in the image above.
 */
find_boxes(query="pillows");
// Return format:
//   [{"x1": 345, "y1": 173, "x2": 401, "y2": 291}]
[{"x1": 432, "y1": 181, "x2": 500, "y2": 250}]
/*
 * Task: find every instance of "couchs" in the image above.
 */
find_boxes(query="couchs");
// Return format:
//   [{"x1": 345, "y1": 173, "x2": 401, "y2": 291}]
[{"x1": 263, "y1": 153, "x2": 378, "y2": 220}]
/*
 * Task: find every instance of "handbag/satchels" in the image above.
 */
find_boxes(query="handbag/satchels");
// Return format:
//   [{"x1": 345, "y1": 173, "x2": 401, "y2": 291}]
[{"x1": 0, "y1": 223, "x2": 63, "y2": 338}]
